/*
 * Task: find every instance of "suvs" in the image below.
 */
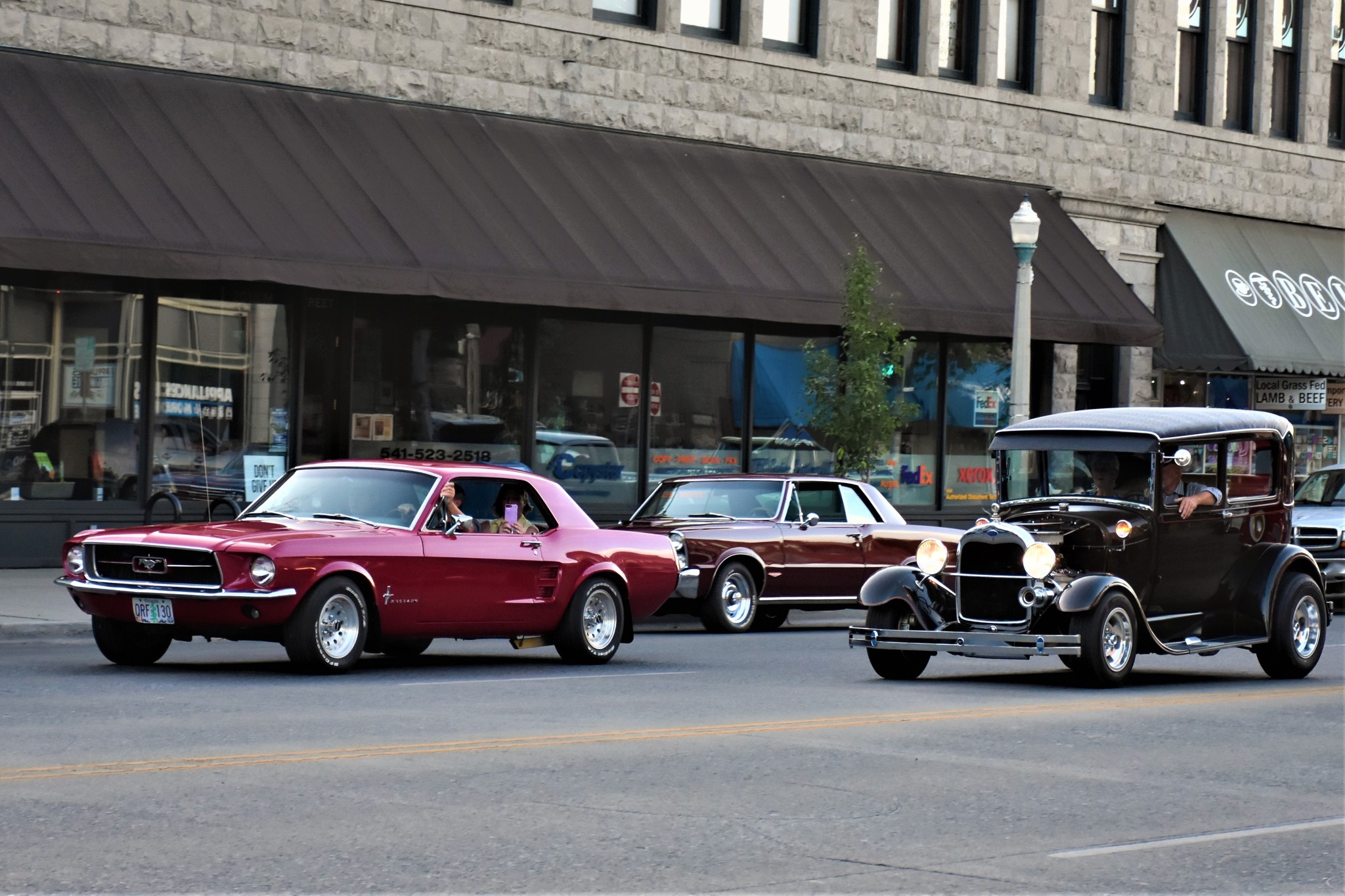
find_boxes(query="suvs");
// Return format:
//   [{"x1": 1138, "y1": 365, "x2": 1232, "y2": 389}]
[{"x1": 846, "y1": 407, "x2": 1333, "y2": 686}]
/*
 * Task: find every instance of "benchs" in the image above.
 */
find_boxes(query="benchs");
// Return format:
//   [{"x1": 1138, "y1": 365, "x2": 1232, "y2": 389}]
[{"x1": 1181, "y1": 473, "x2": 1272, "y2": 498}]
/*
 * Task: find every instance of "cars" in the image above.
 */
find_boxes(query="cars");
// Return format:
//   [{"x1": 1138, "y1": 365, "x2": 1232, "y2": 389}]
[
  {"x1": 1288, "y1": 463, "x2": 1345, "y2": 599},
  {"x1": 595, "y1": 475, "x2": 968, "y2": 635},
  {"x1": 49, "y1": 461, "x2": 680, "y2": 679},
  {"x1": 347, "y1": 410, "x2": 518, "y2": 461},
  {"x1": 536, "y1": 428, "x2": 627, "y2": 507},
  {"x1": 20, "y1": 418, "x2": 286, "y2": 498}
]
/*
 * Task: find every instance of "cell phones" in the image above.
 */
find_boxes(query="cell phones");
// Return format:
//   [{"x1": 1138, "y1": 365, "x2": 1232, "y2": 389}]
[{"x1": 504, "y1": 504, "x2": 518, "y2": 530}]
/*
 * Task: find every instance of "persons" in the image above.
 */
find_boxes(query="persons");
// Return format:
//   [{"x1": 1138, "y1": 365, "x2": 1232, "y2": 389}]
[
  {"x1": 396, "y1": 482, "x2": 482, "y2": 533},
  {"x1": 764, "y1": 495, "x2": 797, "y2": 523},
  {"x1": 1079, "y1": 451, "x2": 1126, "y2": 502},
  {"x1": 1140, "y1": 447, "x2": 1223, "y2": 519},
  {"x1": 1028, "y1": 450, "x2": 1038, "y2": 498},
  {"x1": 479, "y1": 483, "x2": 539, "y2": 536},
  {"x1": 153, "y1": 427, "x2": 176, "y2": 447}
]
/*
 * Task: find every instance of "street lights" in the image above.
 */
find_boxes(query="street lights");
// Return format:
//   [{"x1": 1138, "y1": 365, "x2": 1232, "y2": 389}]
[{"x1": 1004, "y1": 199, "x2": 1042, "y2": 506}]
